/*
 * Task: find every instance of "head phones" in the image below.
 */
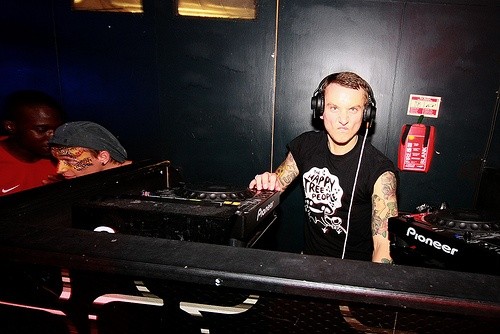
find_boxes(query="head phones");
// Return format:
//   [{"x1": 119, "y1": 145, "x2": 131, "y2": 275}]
[{"x1": 311, "y1": 76, "x2": 376, "y2": 123}]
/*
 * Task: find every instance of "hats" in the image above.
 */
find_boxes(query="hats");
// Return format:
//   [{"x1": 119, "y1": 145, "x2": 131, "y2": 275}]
[{"x1": 49, "y1": 121, "x2": 129, "y2": 164}]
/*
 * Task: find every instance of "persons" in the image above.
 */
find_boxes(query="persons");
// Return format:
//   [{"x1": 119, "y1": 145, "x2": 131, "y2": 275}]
[
  {"x1": 249, "y1": 72, "x2": 398, "y2": 264},
  {"x1": 0, "y1": 96, "x2": 66, "y2": 196},
  {"x1": 49, "y1": 121, "x2": 135, "y2": 180}
]
